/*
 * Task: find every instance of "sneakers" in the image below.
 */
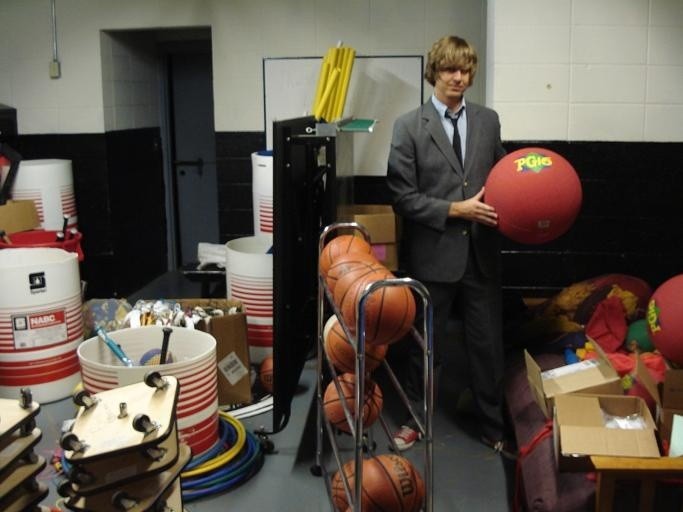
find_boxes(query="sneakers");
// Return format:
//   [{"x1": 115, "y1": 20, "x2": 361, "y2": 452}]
[
  {"x1": 484, "y1": 434, "x2": 518, "y2": 460},
  {"x1": 388, "y1": 421, "x2": 419, "y2": 450}
]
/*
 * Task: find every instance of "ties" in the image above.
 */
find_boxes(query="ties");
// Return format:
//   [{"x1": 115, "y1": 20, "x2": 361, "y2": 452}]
[{"x1": 444, "y1": 111, "x2": 462, "y2": 166}]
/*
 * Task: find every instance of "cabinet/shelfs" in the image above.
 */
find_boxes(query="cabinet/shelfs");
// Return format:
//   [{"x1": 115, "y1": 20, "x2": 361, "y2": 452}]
[{"x1": 310, "y1": 221, "x2": 434, "y2": 512}]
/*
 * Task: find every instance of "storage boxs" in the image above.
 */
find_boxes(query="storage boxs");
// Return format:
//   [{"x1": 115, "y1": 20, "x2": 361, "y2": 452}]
[
  {"x1": 524, "y1": 334, "x2": 683, "y2": 459},
  {"x1": 335, "y1": 205, "x2": 401, "y2": 272},
  {"x1": 127, "y1": 298, "x2": 251, "y2": 405}
]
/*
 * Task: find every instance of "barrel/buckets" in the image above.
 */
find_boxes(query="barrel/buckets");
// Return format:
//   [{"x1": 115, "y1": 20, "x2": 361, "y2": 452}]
[
  {"x1": 1, "y1": 157, "x2": 78, "y2": 231},
  {"x1": 224, "y1": 233, "x2": 275, "y2": 366},
  {"x1": 250, "y1": 152, "x2": 273, "y2": 238},
  {"x1": 0, "y1": 250, "x2": 85, "y2": 404},
  {"x1": 78, "y1": 326, "x2": 219, "y2": 458}
]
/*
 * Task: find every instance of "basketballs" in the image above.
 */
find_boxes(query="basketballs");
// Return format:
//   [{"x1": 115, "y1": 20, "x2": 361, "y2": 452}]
[
  {"x1": 260, "y1": 354, "x2": 273, "y2": 394},
  {"x1": 320, "y1": 236, "x2": 427, "y2": 512}
]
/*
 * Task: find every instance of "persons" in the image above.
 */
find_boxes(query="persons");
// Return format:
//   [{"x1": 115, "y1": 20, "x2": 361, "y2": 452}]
[{"x1": 384, "y1": 34, "x2": 514, "y2": 462}]
